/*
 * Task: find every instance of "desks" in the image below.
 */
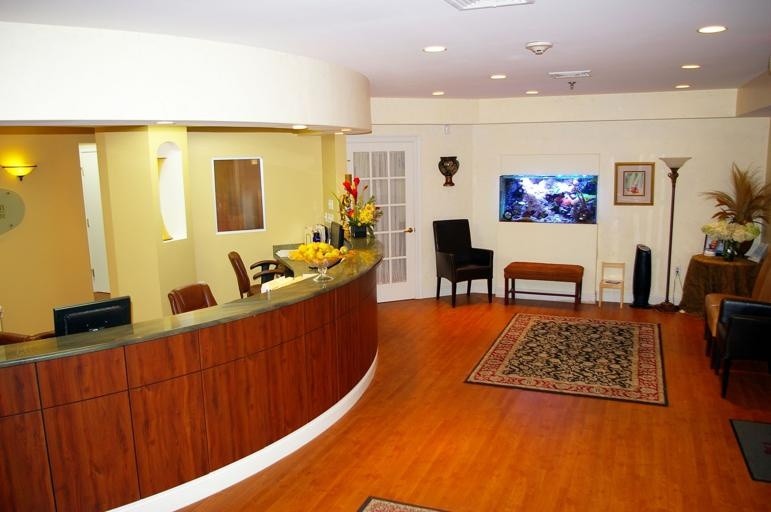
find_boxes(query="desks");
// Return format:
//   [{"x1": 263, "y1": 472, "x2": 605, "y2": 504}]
[{"x1": 680, "y1": 253, "x2": 759, "y2": 311}]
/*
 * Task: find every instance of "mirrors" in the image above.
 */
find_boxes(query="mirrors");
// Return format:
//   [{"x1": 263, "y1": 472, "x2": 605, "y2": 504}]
[{"x1": 210, "y1": 157, "x2": 267, "y2": 235}]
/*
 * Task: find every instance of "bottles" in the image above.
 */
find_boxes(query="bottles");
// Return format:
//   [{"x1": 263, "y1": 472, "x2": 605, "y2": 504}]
[{"x1": 304, "y1": 226, "x2": 320, "y2": 246}]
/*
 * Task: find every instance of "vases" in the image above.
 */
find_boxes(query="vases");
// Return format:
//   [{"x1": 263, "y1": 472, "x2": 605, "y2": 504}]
[
  {"x1": 350, "y1": 225, "x2": 367, "y2": 238},
  {"x1": 723, "y1": 241, "x2": 754, "y2": 255}
]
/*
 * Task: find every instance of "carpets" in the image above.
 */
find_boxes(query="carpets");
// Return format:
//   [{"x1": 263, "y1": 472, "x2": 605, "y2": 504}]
[
  {"x1": 466, "y1": 312, "x2": 668, "y2": 407},
  {"x1": 729, "y1": 418, "x2": 771, "y2": 483},
  {"x1": 355, "y1": 495, "x2": 444, "y2": 512}
]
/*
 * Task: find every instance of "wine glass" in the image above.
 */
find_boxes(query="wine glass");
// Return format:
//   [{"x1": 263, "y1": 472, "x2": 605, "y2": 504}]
[{"x1": 302, "y1": 256, "x2": 344, "y2": 282}]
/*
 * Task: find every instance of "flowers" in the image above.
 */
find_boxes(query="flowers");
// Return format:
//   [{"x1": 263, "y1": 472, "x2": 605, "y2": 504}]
[
  {"x1": 700, "y1": 160, "x2": 771, "y2": 243},
  {"x1": 332, "y1": 176, "x2": 383, "y2": 239}
]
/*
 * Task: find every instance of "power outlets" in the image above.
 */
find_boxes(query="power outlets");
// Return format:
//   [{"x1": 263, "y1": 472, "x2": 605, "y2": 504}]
[{"x1": 673, "y1": 265, "x2": 684, "y2": 278}]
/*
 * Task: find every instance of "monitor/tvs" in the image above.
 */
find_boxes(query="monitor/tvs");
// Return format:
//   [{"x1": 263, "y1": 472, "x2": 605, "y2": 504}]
[
  {"x1": 330, "y1": 222, "x2": 344, "y2": 248},
  {"x1": 51, "y1": 296, "x2": 133, "y2": 336}
]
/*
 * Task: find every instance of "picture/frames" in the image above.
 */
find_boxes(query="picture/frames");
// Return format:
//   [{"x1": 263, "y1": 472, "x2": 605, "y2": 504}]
[
  {"x1": 704, "y1": 233, "x2": 725, "y2": 252},
  {"x1": 614, "y1": 163, "x2": 655, "y2": 205}
]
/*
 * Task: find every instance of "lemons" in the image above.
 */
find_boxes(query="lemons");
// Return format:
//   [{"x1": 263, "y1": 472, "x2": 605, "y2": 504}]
[{"x1": 299, "y1": 242, "x2": 348, "y2": 261}]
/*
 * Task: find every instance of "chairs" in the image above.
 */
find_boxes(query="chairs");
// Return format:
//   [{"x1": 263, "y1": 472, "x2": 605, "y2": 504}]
[
  {"x1": 599, "y1": 262, "x2": 626, "y2": 308},
  {"x1": 433, "y1": 219, "x2": 494, "y2": 307},
  {"x1": 704, "y1": 244, "x2": 771, "y2": 398},
  {"x1": 169, "y1": 283, "x2": 218, "y2": 314},
  {"x1": 228, "y1": 251, "x2": 284, "y2": 297}
]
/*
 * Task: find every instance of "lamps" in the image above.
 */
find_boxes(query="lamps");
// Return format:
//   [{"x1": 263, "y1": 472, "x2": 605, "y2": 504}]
[
  {"x1": 1, "y1": 164, "x2": 37, "y2": 182},
  {"x1": 439, "y1": 157, "x2": 459, "y2": 187},
  {"x1": 659, "y1": 156, "x2": 692, "y2": 312}
]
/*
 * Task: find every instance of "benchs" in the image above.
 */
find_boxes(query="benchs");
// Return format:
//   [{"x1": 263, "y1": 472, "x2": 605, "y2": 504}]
[{"x1": 504, "y1": 261, "x2": 583, "y2": 311}]
